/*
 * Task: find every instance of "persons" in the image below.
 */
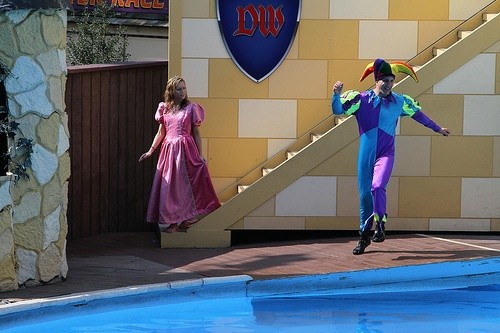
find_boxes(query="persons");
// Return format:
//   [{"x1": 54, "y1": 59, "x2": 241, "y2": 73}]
[
  {"x1": 138, "y1": 76, "x2": 211, "y2": 235},
  {"x1": 332, "y1": 59, "x2": 451, "y2": 254}
]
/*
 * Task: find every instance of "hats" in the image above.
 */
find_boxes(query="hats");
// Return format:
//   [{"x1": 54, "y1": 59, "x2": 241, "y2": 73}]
[{"x1": 359, "y1": 58, "x2": 419, "y2": 83}]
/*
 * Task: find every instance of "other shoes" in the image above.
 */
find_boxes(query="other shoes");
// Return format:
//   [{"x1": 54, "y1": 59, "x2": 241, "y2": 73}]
[
  {"x1": 178, "y1": 221, "x2": 192, "y2": 231},
  {"x1": 166, "y1": 223, "x2": 178, "y2": 232}
]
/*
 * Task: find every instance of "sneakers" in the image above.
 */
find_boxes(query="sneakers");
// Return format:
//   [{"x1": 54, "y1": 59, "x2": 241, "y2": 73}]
[
  {"x1": 372, "y1": 213, "x2": 387, "y2": 242},
  {"x1": 353, "y1": 231, "x2": 371, "y2": 255}
]
